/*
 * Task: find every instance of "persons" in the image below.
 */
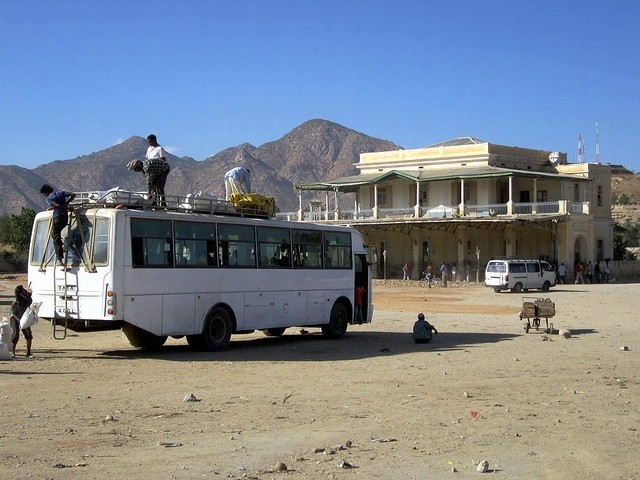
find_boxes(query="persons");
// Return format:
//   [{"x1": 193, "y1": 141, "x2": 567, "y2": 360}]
[
  {"x1": 355, "y1": 281, "x2": 366, "y2": 323},
  {"x1": 439, "y1": 261, "x2": 448, "y2": 287},
  {"x1": 40, "y1": 184, "x2": 76, "y2": 266},
  {"x1": 224, "y1": 166, "x2": 251, "y2": 206},
  {"x1": 574, "y1": 259, "x2": 584, "y2": 284},
  {"x1": 425, "y1": 262, "x2": 433, "y2": 288},
  {"x1": 579, "y1": 261, "x2": 584, "y2": 284},
  {"x1": 412, "y1": 313, "x2": 438, "y2": 343},
  {"x1": 595, "y1": 260, "x2": 601, "y2": 284},
  {"x1": 558, "y1": 262, "x2": 566, "y2": 284},
  {"x1": 604, "y1": 260, "x2": 610, "y2": 283},
  {"x1": 465, "y1": 261, "x2": 471, "y2": 282},
  {"x1": 402, "y1": 262, "x2": 410, "y2": 282},
  {"x1": 452, "y1": 263, "x2": 457, "y2": 282},
  {"x1": 126, "y1": 158, "x2": 170, "y2": 208},
  {"x1": 145, "y1": 134, "x2": 166, "y2": 209},
  {"x1": 586, "y1": 261, "x2": 594, "y2": 283},
  {"x1": 9, "y1": 285, "x2": 35, "y2": 358}
]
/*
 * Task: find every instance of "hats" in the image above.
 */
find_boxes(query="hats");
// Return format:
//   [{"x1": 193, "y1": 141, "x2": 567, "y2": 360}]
[{"x1": 127, "y1": 159, "x2": 141, "y2": 171}]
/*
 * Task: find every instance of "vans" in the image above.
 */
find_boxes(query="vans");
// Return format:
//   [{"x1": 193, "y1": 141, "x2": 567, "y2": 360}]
[{"x1": 485, "y1": 257, "x2": 557, "y2": 293}]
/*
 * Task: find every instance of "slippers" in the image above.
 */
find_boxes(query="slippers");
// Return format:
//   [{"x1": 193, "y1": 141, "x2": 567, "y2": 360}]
[
  {"x1": 10, "y1": 352, "x2": 14, "y2": 357},
  {"x1": 26, "y1": 355, "x2": 34, "y2": 359}
]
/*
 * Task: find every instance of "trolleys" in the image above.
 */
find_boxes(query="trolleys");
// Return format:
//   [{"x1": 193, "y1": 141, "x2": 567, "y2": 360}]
[{"x1": 518, "y1": 297, "x2": 555, "y2": 334}]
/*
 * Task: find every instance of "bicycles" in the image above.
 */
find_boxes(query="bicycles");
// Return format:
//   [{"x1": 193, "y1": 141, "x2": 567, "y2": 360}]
[{"x1": 420, "y1": 272, "x2": 442, "y2": 287}]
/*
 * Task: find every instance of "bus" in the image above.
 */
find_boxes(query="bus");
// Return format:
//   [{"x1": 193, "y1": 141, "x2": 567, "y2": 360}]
[{"x1": 28, "y1": 188, "x2": 378, "y2": 351}]
